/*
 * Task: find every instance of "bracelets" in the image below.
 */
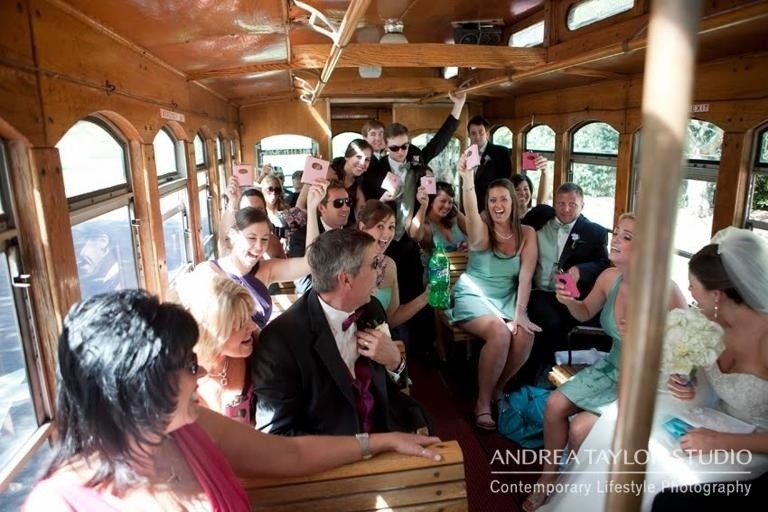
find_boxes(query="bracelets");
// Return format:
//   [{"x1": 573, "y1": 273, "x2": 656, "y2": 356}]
[{"x1": 354, "y1": 433, "x2": 375, "y2": 461}]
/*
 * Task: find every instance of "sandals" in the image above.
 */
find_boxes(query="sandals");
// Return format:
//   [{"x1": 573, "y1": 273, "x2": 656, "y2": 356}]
[{"x1": 521, "y1": 475, "x2": 558, "y2": 512}]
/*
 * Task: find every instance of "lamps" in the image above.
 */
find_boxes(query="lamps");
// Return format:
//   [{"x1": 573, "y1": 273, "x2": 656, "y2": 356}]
[
  {"x1": 375, "y1": 0, "x2": 416, "y2": 44},
  {"x1": 357, "y1": 64, "x2": 382, "y2": 80}
]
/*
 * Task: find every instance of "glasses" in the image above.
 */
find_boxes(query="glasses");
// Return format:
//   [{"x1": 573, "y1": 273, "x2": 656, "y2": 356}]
[
  {"x1": 388, "y1": 143, "x2": 410, "y2": 153},
  {"x1": 264, "y1": 186, "x2": 281, "y2": 195},
  {"x1": 343, "y1": 256, "x2": 378, "y2": 274},
  {"x1": 326, "y1": 198, "x2": 353, "y2": 208},
  {"x1": 166, "y1": 352, "x2": 198, "y2": 376}
]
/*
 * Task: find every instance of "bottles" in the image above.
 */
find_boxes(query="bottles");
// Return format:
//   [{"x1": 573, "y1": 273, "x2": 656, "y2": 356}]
[{"x1": 427, "y1": 241, "x2": 451, "y2": 308}]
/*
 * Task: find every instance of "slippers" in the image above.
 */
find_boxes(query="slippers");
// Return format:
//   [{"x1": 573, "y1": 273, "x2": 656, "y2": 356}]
[{"x1": 474, "y1": 406, "x2": 496, "y2": 431}]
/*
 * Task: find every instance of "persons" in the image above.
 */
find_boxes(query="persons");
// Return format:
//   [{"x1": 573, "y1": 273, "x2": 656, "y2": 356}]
[
  {"x1": 14, "y1": 289, "x2": 447, "y2": 512},
  {"x1": 165, "y1": 273, "x2": 259, "y2": 432},
  {"x1": 531, "y1": 222, "x2": 767, "y2": 512},
  {"x1": 520, "y1": 213, "x2": 689, "y2": 512},
  {"x1": 191, "y1": 89, "x2": 609, "y2": 441}
]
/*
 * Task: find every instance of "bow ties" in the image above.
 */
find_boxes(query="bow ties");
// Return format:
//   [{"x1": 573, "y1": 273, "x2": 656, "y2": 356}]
[
  {"x1": 398, "y1": 161, "x2": 410, "y2": 170},
  {"x1": 342, "y1": 309, "x2": 364, "y2": 332},
  {"x1": 553, "y1": 223, "x2": 569, "y2": 233}
]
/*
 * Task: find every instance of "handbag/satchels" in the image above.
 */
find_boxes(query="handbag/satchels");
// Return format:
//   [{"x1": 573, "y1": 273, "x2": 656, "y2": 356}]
[{"x1": 497, "y1": 385, "x2": 554, "y2": 449}]
